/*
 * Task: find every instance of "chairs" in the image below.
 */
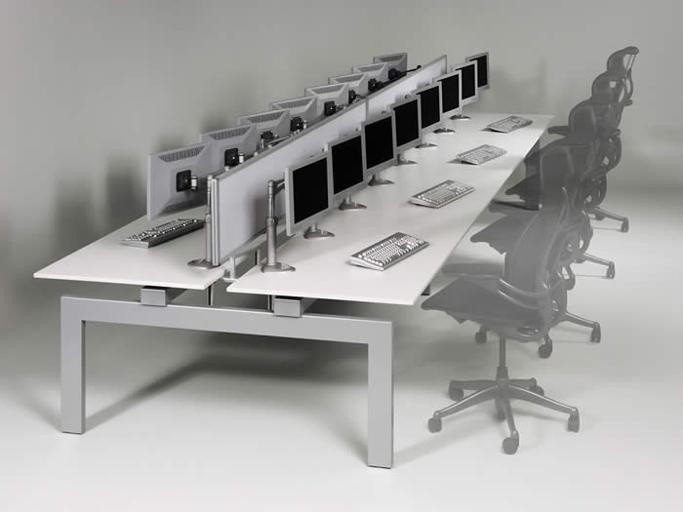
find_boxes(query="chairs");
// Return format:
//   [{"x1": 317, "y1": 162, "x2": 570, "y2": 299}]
[
  {"x1": 475, "y1": 148, "x2": 603, "y2": 357},
  {"x1": 421, "y1": 185, "x2": 580, "y2": 455},
  {"x1": 470, "y1": 45, "x2": 639, "y2": 289}
]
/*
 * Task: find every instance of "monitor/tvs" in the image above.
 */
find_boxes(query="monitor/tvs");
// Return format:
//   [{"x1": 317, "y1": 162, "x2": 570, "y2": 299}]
[
  {"x1": 262, "y1": 151, "x2": 334, "y2": 273},
  {"x1": 270, "y1": 95, "x2": 319, "y2": 136},
  {"x1": 339, "y1": 110, "x2": 398, "y2": 212},
  {"x1": 145, "y1": 138, "x2": 226, "y2": 270},
  {"x1": 368, "y1": 94, "x2": 423, "y2": 186},
  {"x1": 236, "y1": 107, "x2": 292, "y2": 152},
  {"x1": 372, "y1": 52, "x2": 408, "y2": 82},
  {"x1": 415, "y1": 69, "x2": 462, "y2": 149},
  {"x1": 352, "y1": 62, "x2": 391, "y2": 95},
  {"x1": 434, "y1": 60, "x2": 479, "y2": 134},
  {"x1": 303, "y1": 81, "x2": 350, "y2": 121},
  {"x1": 450, "y1": 51, "x2": 490, "y2": 121},
  {"x1": 396, "y1": 80, "x2": 443, "y2": 166},
  {"x1": 305, "y1": 130, "x2": 368, "y2": 238},
  {"x1": 327, "y1": 71, "x2": 369, "y2": 106},
  {"x1": 198, "y1": 122, "x2": 260, "y2": 170}
]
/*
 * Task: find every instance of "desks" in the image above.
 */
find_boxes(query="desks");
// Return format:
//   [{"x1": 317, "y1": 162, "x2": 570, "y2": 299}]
[{"x1": 31, "y1": 113, "x2": 553, "y2": 470}]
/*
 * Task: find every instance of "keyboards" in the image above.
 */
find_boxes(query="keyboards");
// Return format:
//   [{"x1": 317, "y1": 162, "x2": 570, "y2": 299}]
[
  {"x1": 409, "y1": 178, "x2": 477, "y2": 209},
  {"x1": 120, "y1": 218, "x2": 204, "y2": 248},
  {"x1": 487, "y1": 116, "x2": 532, "y2": 133},
  {"x1": 349, "y1": 232, "x2": 429, "y2": 270},
  {"x1": 456, "y1": 145, "x2": 506, "y2": 165}
]
